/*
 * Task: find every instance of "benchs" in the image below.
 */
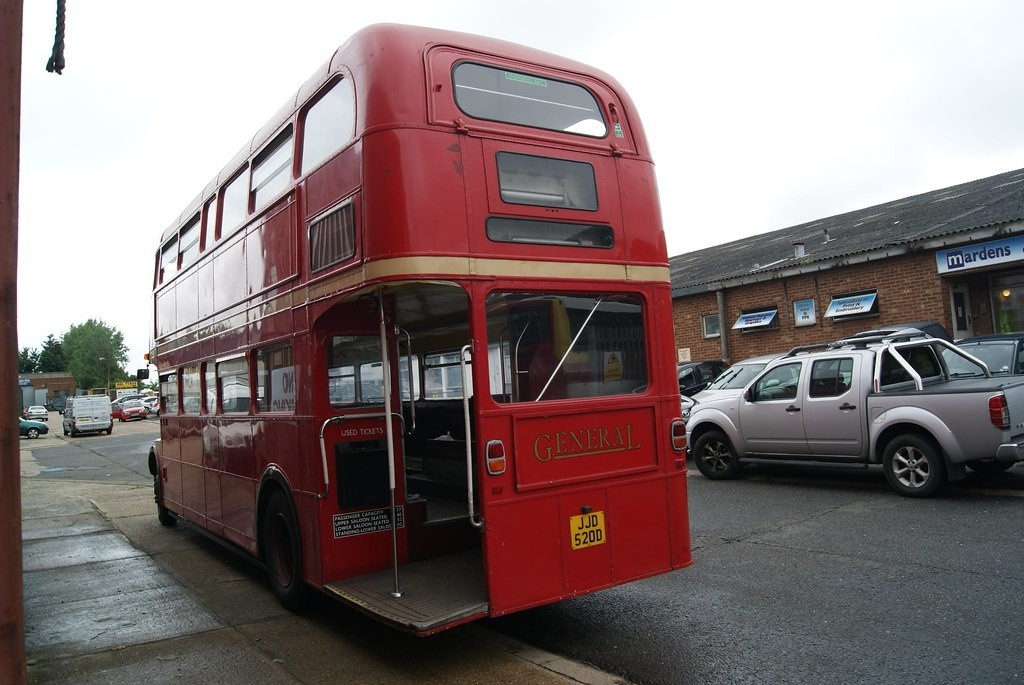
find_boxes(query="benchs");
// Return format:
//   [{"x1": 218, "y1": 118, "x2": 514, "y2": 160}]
[{"x1": 401, "y1": 401, "x2": 477, "y2": 472}]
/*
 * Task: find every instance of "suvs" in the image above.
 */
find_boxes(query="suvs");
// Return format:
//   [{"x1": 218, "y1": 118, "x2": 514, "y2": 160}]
[
  {"x1": 329, "y1": 381, "x2": 384, "y2": 402},
  {"x1": 676, "y1": 360, "x2": 731, "y2": 396}
]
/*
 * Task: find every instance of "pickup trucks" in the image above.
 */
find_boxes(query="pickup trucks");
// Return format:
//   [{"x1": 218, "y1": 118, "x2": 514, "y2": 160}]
[{"x1": 685, "y1": 327, "x2": 1024, "y2": 498}]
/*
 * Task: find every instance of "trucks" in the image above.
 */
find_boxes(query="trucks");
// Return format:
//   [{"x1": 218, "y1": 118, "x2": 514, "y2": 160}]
[{"x1": 59, "y1": 395, "x2": 114, "y2": 438}]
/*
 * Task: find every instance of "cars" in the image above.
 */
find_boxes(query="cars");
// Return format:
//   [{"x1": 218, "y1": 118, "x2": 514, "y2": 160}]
[
  {"x1": 111, "y1": 394, "x2": 200, "y2": 422},
  {"x1": 27, "y1": 405, "x2": 48, "y2": 421},
  {"x1": 18, "y1": 417, "x2": 49, "y2": 439},
  {"x1": 689, "y1": 321, "x2": 1023, "y2": 409}
]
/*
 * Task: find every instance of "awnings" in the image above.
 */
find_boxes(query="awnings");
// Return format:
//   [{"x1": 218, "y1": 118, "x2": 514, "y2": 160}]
[
  {"x1": 825, "y1": 294, "x2": 875, "y2": 318},
  {"x1": 732, "y1": 310, "x2": 778, "y2": 330}
]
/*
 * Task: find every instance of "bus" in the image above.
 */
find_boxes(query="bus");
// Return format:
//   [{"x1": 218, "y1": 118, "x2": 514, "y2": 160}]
[{"x1": 137, "y1": 23, "x2": 693, "y2": 638}]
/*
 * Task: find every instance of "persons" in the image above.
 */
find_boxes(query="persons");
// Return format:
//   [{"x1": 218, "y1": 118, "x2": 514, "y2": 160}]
[{"x1": 530, "y1": 309, "x2": 582, "y2": 401}]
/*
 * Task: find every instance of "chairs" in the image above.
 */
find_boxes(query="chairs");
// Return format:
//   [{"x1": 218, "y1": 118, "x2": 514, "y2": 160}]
[{"x1": 814, "y1": 369, "x2": 847, "y2": 398}]
